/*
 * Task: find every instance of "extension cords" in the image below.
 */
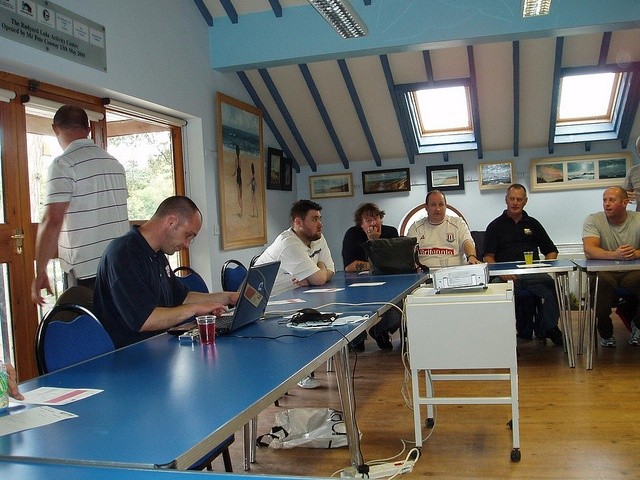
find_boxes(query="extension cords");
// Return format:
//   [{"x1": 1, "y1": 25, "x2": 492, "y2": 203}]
[{"x1": 340, "y1": 459, "x2": 413, "y2": 479}]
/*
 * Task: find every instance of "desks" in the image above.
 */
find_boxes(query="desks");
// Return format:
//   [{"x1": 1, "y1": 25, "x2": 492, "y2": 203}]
[
  {"x1": 571, "y1": 258, "x2": 640, "y2": 370},
  {"x1": 418, "y1": 259, "x2": 577, "y2": 368},
  {"x1": 405, "y1": 279, "x2": 521, "y2": 461},
  {"x1": 0, "y1": 271, "x2": 429, "y2": 479}
]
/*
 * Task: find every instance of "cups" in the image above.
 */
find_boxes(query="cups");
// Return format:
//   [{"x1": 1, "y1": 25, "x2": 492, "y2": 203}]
[
  {"x1": 524, "y1": 252, "x2": 534, "y2": 265},
  {"x1": 196, "y1": 316, "x2": 218, "y2": 344}
]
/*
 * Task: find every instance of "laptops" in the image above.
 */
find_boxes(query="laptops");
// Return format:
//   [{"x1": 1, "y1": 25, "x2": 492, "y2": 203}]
[{"x1": 167, "y1": 261, "x2": 281, "y2": 335}]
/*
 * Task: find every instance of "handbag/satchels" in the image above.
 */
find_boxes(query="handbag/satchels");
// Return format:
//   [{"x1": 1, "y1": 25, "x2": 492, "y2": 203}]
[
  {"x1": 256, "y1": 407, "x2": 364, "y2": 450},
  {"x1": 364, "y1": 237, "x2": 430, "y2": 275}
]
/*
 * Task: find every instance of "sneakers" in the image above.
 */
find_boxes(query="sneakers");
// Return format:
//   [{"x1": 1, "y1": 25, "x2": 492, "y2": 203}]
[
  {"x1": 297, "y1": 376, "x2": 321, "y2": 389},
  {"x1": 547, "y1": 325, "x2": 566, "y2": 345},
  {"x1": 628, "y1": 320, "x2": 640, "y2": 346},
  {"x1": 600, "y1": 334, "x2": 616, "y2": 347},
  {"x1": 368, "y1": 325, "x2": 393, "y2": 352},
  {"x1": 349, "y1": 342, "x2": 365, "y2": 352}
]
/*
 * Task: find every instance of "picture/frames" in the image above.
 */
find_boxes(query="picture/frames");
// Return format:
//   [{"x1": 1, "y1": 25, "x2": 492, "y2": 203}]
[
  {"x1": 479, "y1": 160, "x2": 515, "y2": 190},
  {"x1": 362, "y1": 167, "x2": 410, "y2": 194},
  {"x1": 282, "y1": 157, "x2": 292, "y2": 191},
  {"x1": 215, "y1": 91, "x2": 268, "y2": 252},
  {"x1": 426, "y1": 163, "x2": 465, "y2": 192},
  {"x1": 530, "y1": 152, "x2": 632, "y2": 192},
  {"x1": 267, "y1": 147, "x2": 283, "y2": 190},
  {"x1": 309, "y1": 173, "x2": 354, "y2": 199}
]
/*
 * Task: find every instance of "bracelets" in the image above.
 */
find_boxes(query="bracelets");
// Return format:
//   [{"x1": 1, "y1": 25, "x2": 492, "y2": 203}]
[{"x1": 467, "y1": 255, "x2": 478, "y2": 259}]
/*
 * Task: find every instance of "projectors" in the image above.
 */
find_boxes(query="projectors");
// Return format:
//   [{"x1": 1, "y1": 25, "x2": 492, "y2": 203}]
[{"x1": 433, "y1": 262, "x2": 489, "y2": 293}]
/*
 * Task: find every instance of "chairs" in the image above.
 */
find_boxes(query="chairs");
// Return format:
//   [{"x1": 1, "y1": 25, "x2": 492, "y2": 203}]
[
  {"x1": 221, "y1": 260, "x2": 288, "y2": 406},
  {"x1": 174, "y1": 267, "x2": 209, "y2": 294},
  {"x1": 55, "y1": 287, "x2": 97, "y2": 311},
  {"x1": 34, "y1": 303, "x2": 235, "y2": 473}
]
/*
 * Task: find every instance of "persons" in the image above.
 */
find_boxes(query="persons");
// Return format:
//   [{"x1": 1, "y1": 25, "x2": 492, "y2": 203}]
[
  {"x1": 483, "y1": 184, "x2": 569, "y2": 345},
  {"x1": 94, "y1": 195, "x2": 240, "y2": 349},
  {"x1": 582, "y1": 186, "x2": 639, "y2": 348},
  {"x1": 30, "y1": 105, "x2": 129, "y2": 307},
  {"x1": 3, "y1": 362, "x2": 24, "y2": 401},
  {"x1": 406, "y1": 190, "x2": 483, "y2": 283},
  {"x1": 620, "y1": 138, "x2": 640, "y2": 211},
  {"x1": 253, "y1": 200, "x2": 335, "y2": 389},
  {"x1": 342, "y1": 202, "x2": 402, "y2": 351}
]
